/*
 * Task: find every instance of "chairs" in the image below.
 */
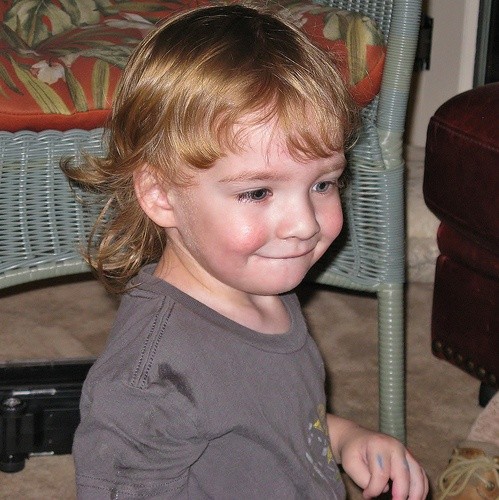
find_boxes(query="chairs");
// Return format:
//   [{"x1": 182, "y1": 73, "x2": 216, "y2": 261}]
[{"x1": 1, "y1": 0, "x2": 423, "y2": 447}]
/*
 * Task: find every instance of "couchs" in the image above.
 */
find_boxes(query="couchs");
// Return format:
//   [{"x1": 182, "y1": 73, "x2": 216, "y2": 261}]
[{"x1": 424, "y1": 80, "x2": 499, "y2": 407}]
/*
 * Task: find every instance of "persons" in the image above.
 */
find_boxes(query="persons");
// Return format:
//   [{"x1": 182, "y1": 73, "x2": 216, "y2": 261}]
[{"x1": 56, "y1": 4, "x2": 431, "y2": 500}]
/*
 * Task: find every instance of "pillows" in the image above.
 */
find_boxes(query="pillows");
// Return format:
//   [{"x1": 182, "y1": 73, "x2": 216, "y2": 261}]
[{"x1": 1, "y1": 0, "x2": 385, "y2": 134}]
